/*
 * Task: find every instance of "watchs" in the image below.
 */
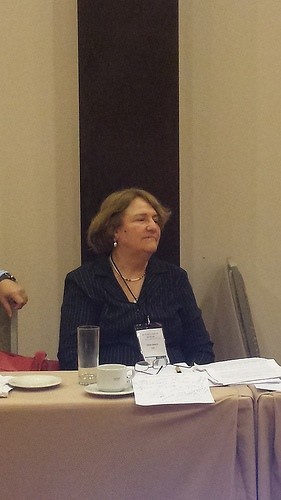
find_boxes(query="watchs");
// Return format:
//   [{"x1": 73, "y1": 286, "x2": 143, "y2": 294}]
[{"x1": 0, "y1": 272, "x2": 16, "y2": 283}]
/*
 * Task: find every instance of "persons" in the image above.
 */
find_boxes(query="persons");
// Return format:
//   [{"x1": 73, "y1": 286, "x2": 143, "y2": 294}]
[
  {"x1": 57, "y1": 189, "x2": 215, "y2": 372},
  {"x1": 0, "y1": 267, "x2": 29, "y2": 318}
]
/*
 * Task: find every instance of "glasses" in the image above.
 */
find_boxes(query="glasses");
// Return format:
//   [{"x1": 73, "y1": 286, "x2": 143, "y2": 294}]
[{"x1": 134, "y1": 358, "x2": 167, "y2": 375}]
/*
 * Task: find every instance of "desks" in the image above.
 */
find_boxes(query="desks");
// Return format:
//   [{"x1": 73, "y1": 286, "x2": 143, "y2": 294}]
[{"x1": 1, "y1": 371, "x2": 281, "y2": 500}]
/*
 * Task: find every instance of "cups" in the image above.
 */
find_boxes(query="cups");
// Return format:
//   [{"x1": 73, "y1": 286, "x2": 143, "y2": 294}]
[
  {"x1": 97, "y1": 364, "x2": 135, "y2": 392},
  {"x1": 77, "y1": 325, "x2": 99, "y2": 386}
]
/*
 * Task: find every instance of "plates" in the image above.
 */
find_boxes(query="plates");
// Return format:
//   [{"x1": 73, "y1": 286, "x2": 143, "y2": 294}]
[
  {"x1": 9, "y1": 374, "x2": 62, "y2": 388},
  {"x1": 84, "y1": 384, "x2": 134, "y2": 396}
]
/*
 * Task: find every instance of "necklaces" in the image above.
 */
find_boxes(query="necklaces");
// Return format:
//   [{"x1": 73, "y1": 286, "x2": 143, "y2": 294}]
[{"x1": 109, "y1": 254, "x2": 146, "y2": 282}]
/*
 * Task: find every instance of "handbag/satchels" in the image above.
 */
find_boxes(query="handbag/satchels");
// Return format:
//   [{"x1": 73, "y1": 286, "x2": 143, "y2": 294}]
[{"x1": 0, "y1": 349, "x2": 61, "y2": 372}]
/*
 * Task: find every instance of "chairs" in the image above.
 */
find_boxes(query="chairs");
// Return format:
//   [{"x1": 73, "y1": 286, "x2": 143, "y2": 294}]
[
  {"x1": 227, "y1": 257, "x2": 261, "y2": 358},
  {"x1": 0, "y1": 304, "x2": 18, "y2": 354}
]
